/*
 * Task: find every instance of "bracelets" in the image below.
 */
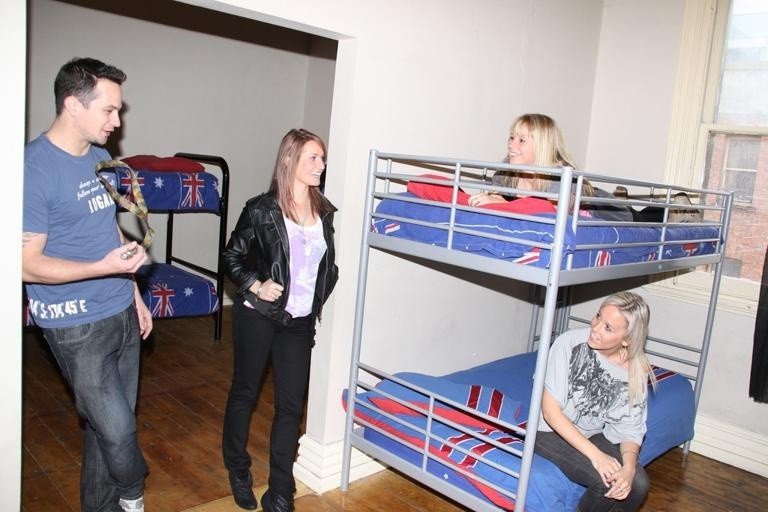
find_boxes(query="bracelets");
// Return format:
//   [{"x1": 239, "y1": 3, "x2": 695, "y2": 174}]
[
  {"x1": 620, "y1": 450, "x2": 639, "y2": 457},
  {"x1": 256, "y1": 283, "x2": 263, "y2": 298}
]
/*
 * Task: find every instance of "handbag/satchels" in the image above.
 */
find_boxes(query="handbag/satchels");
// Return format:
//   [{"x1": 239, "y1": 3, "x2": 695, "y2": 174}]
[{"x1": 115, "y1": 203, "x2": 156, "y2": 252}]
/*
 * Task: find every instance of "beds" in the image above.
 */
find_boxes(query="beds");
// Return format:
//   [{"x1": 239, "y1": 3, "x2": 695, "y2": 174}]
[
  {"x1": 22, "y1": 153, "x2": 229, "y2": 341},
  {"x1": 340, "y1": 149, "x2": 735, "y2": 512}
]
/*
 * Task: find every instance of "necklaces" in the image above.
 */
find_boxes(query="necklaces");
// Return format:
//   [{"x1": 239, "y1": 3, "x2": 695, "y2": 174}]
[
  {"x1": 298, "y1": 198, "x2": 310, "y2": 227},
  {"x1": 525, "y1": 177, "x2": 536, "y2": 190}
]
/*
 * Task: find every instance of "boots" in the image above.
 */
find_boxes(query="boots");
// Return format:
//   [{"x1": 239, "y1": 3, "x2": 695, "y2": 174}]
[
  {"x1": 665, "y1": 193, "x2": 702, "y2": 223},
  {"x1": 613, "y1": 186, "x2": 631, "y2": 208}
]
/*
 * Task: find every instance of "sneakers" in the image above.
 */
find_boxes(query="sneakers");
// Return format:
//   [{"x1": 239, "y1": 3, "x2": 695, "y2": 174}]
[
  {"x1": 228, "y1": 470, "x2": 257, "y2": 510},
  {"x1": 118, "y1": 496, "x2": 146, "y2": 512},
  {"x1": 261, "y1": 487, "x2": 296, "y2": 511}
]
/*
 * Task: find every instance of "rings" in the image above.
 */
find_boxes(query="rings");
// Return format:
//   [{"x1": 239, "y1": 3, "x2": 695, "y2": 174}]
[{"x1": 475, "y1": 197, "x2": 480, "y2": 203}]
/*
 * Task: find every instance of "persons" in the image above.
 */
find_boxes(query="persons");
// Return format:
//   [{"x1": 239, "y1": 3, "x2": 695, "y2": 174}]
[
  {"x1": 532, "y1": 291, "x2": 659, "y2": 511},
  {"x1": 467, "y1": 113, "x2": 703, "y2": 225},
  {"x1": 22, "y1": 58, "x2": 154, "y2": 511},
  {"x1": 221, "y1": 128, "x2": 339, "y2": 512}
]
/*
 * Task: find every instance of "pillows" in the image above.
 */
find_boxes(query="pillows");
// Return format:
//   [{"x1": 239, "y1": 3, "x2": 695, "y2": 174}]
[
  {"x1": 368, "y1": 372, "x2": 522, "y2": 430},
  {"x1": 407, "y1": 174, "x2": 557, "y2": 216},
  {"x1": 144, "y1": 157, "x2": 206, "y2": 173},
  {"x1": 120, "y1": 154, "x2": 160, "y2": 171}
]
